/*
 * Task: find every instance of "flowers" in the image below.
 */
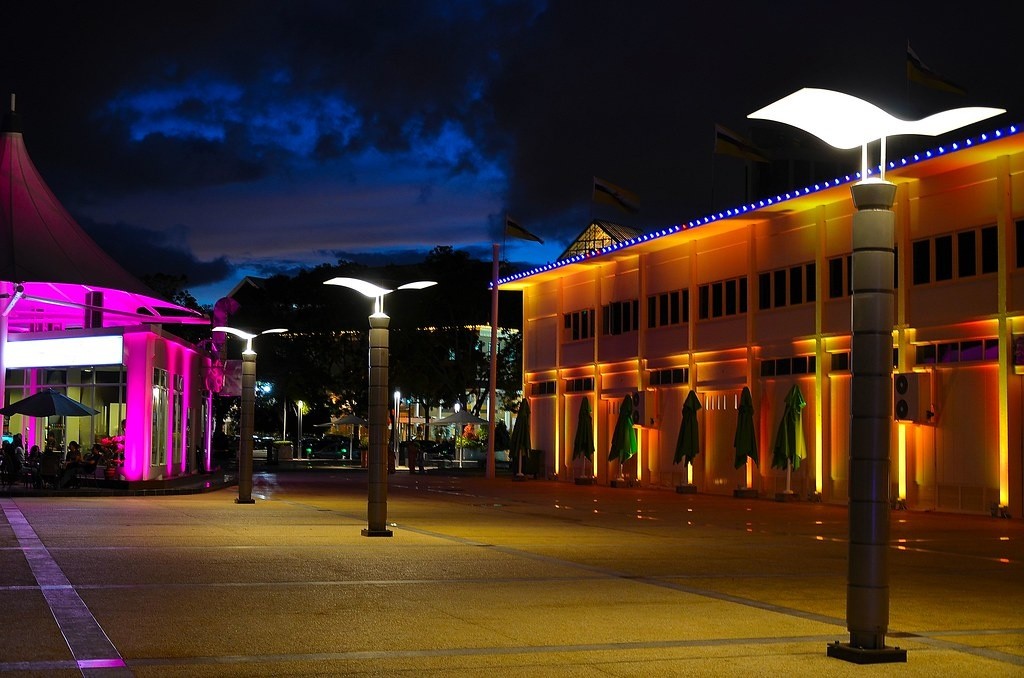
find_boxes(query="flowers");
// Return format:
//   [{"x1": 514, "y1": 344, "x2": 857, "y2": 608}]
[
  {"x1": 463, "y1": 424, "x2": 479, "y2": 440},
  {"x1": 100, "y1": 436, "x2": 124, "y2": 468}
]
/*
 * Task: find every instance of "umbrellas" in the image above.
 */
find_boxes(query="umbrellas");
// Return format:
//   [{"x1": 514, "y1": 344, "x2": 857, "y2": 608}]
[
  {"x1": 432, "y1": 409, "x2": 488, "y2": 468},
  {"x1": 314, "y1": 421, "x2": 341, "y2": 435},
  {"x1": 0, "y1": 388, "x2": 101, "y2": 448},
  {"x1": 334, "y1": 415, "x2": 367, "y2": 460}
]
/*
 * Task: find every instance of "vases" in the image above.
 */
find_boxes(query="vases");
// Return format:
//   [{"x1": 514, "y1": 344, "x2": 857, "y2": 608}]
[{"x1": 104, "y1": 470, "x2": 115, "y2": 480}]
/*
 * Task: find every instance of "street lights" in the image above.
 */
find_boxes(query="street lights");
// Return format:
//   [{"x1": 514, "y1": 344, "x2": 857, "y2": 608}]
[
  {"x1": 744, "y1": 84, "x2": 1009, "y2": 664},
  {"x1": 211, "y1": 325, "x2": 291, "y2": 505},
  {"x1": 322, "y1": 274, "x2": 440, "y2": 540}
]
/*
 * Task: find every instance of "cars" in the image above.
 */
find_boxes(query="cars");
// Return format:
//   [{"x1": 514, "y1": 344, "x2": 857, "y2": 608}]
[{"x1": 252, "y1": 433, "x2": 362, "y2": 467}]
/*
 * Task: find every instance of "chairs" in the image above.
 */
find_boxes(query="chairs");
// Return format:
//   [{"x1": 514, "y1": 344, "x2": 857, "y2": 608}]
[{"x1": 0, "y1": 452, "x2": 101, "y2": 493}]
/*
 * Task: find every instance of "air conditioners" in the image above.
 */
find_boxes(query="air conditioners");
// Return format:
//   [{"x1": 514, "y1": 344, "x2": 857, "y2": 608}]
[{"x1": 894, "y1": 373, "x2": 931, "y2": 423}]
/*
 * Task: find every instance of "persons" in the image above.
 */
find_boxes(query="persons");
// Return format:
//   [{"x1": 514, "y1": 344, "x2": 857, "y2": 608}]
[
  {"x1": 122, "y1": 419, "x2": 126, "y2": 431},
  {"x1": 65, "y1": 441, "x2": 81, "y2": 464},
  {"x1": 55, "y1": 444, "x2": 100, "y2": 489},
  {"x1": 0, "y1": 429, "x2": 57, "y2": 475}
]
